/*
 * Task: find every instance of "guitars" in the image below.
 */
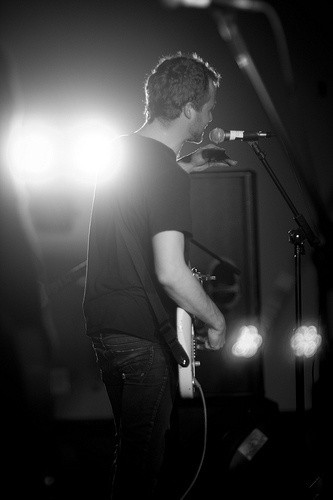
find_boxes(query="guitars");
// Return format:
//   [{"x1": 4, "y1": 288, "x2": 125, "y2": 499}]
[{"x1": 176, "y1": 268, "x2": 217, "y2": 399}]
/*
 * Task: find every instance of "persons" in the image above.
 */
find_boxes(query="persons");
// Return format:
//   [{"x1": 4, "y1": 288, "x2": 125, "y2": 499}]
[{"x1": 82, "y1": 51, "x2": 228, "y2": 500}]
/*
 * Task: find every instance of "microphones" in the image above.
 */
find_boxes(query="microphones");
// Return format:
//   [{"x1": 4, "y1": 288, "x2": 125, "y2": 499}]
[{"x1": 208, "y1": 127, "x2": 278, "y2": 145}]
